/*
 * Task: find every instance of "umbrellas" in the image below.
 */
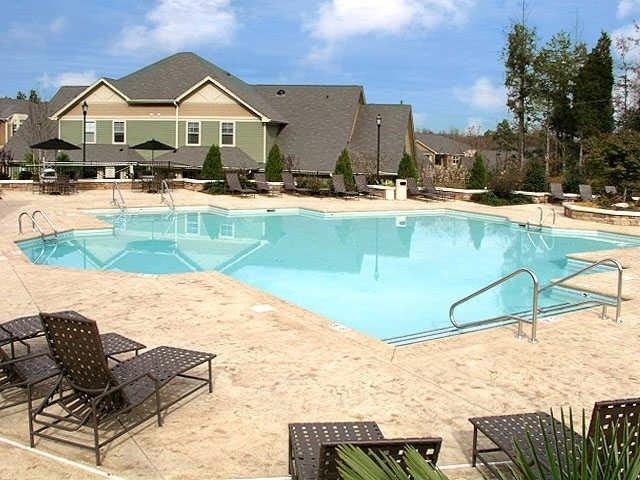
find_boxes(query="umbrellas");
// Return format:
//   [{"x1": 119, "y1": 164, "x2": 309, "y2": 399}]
[
  {"x1": 33, "y1": 244, "x2": 82, "y2": 260},
  {"x1": 125, "y1": 221, "x2": 178, "y2": 253},
  {"x1": 128, "y1": 138, "x2": 179, "y2": 174},
  {"x1": 29, "y1": 137, "x2": 80, "y2": 169}
]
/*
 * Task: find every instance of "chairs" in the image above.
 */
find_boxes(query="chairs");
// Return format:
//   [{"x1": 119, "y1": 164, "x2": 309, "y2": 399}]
[
  {"x1": 406, "y1": 176, "x2": 456, "y2": 202},
  {"x1": 131, "y1": 170, "x2": 173, "y2": 192},
  {"x1": 224, "y1": 170, "x2": 303, "y2": 198},
  {"x1": 287, "y1": 422, "x2": 442, "y2": 480},
  {"x1": 329, "y1": 174, "x2": 375, "y2": 201},
  {"x1": 0, "y1": 309, "x2": 217, "y2": 467},
  {"x1": 468, "y1": 397, "x2": 640, "y2": 480},
  {"x1": 32, "y1": 174, "x2": 78, "y2": 195},
  {"x1": 543, "y1": 182, "x2": 617, "y2": 202}
]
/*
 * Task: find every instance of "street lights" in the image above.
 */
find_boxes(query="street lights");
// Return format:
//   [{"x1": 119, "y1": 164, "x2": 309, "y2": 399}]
[
  {"x1": 375, "y1": 113, "x2": 382, "y2": 184},
  {"x1": 82, "y1": 101, "x2": 88, "y2": 177}
]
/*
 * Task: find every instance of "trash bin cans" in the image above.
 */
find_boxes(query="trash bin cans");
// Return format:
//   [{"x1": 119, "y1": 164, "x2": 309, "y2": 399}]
[{"x1": 395, "y1": 179, "x2": 408, "y2": 201}]
